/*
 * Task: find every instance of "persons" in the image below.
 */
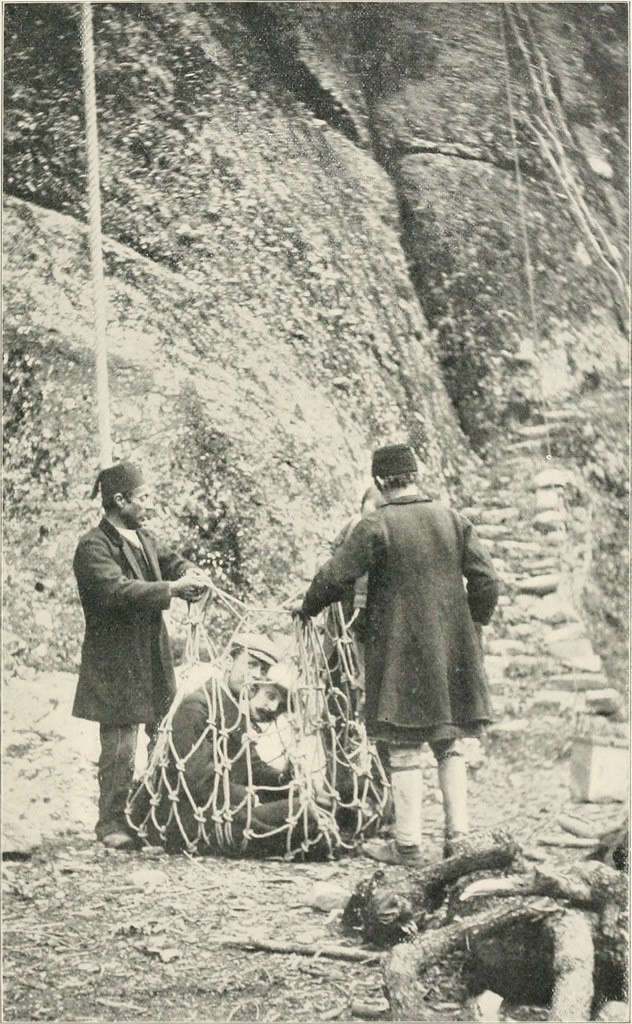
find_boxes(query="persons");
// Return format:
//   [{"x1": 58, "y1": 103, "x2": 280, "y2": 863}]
[
  {"x1": 292, "y1": 443, "x2": 499, "y2": 866},
  {"x1": 71, "y1": 464, "x2": 213, "y2": 845},
  {"x1": 155, "y1": 634, "x2": 392, "y2": 861}
]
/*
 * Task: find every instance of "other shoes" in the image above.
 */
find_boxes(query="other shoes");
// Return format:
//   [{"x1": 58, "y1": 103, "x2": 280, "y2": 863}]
[
  {"x1": 360, "y1": 837, "x2": 425, "y2": 868},
  {"x1": 102, "y1": 830, "x2": 133, "y2": 849}
]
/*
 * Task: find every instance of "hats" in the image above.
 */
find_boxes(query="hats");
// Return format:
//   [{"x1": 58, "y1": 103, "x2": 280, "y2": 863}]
[
  {"x1": 90, "y1": 462, "x2": 146, "y2": 502},
  {"x1": 371, "y1": 444, "x2": 418, "y2": 476},
  {"x1": 267, "y1": 661, "x2": 306, "y2": 705},
  {"x1": 230, "y1": 632, "x2": 280, "y2": 667}
]
